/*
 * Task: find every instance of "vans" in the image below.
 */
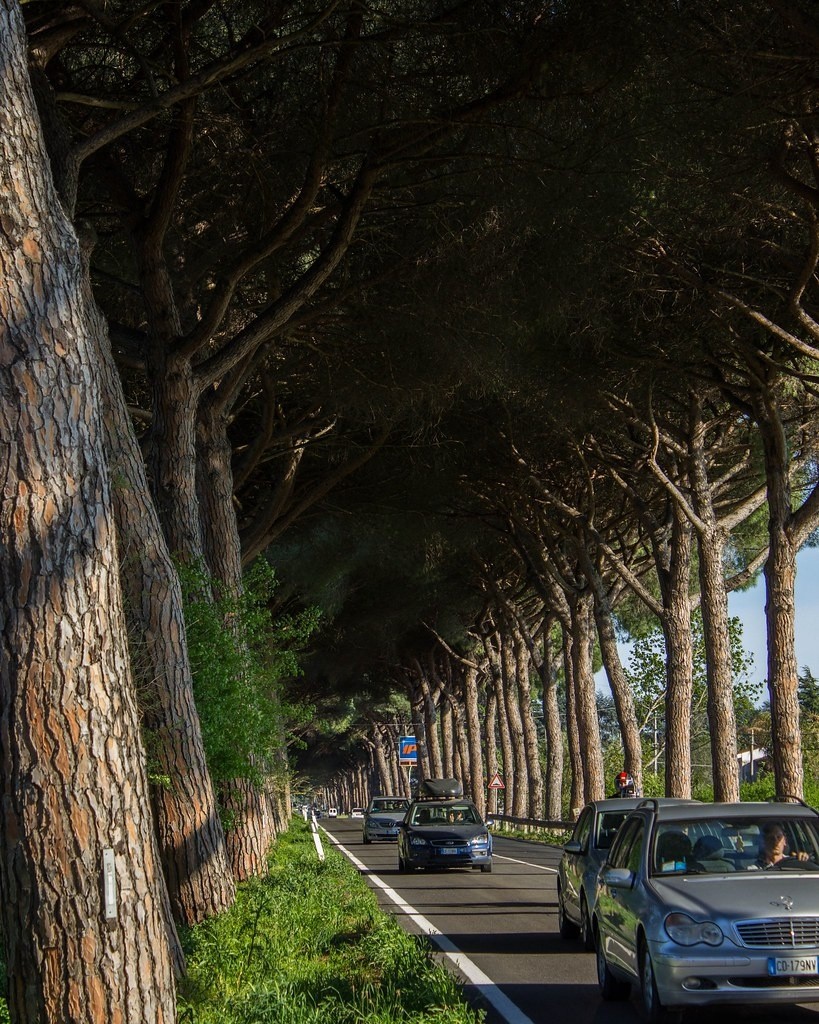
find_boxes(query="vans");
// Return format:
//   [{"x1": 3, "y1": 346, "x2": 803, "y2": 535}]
[{"x1": 328, "y1": 808, "x2": 337, "y2": 818}]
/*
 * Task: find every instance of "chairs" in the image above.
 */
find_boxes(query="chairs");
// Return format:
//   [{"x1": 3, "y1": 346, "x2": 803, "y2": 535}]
[
  {"x1": 599, "y1": 813, "x2": 627, "y2": 849},
  {"x1": 659, "y1": 831, "x2": 706, "y2": 873},
  {"x1": 695, "y1": 835, "x2": 737, "y2": 872},
  {"x1": 416, "y1": 810, "x2": 433, "y2": 823}
]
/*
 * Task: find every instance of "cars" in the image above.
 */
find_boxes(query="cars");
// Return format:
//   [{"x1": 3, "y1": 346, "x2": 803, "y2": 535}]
[
  {"x1": 362, "y1": 796, "x2": 414, "y2": 846},
  {"x1": 555, "y1": 797, "x2": 738, "y2": 948},
  {"x1": 394, "y1": 794, "x2": 494, "y2": 874},
  {"x1": 593, "y1": 794, "x2": 817, "y2": 1024},
  {"x1": 349, "y1": 807, "x2": 365, "y2": 819}
]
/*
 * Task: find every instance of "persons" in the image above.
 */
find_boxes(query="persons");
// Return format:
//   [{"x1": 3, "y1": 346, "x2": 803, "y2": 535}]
[
  {"x1": 607, "y1": 772, "x2": 636, "y2": 799},
  {"x1": 446, "y1": 811, "x2": 464, "y2": 823},
  {"x1": 757, "y1": 823, "x2": 811, "y2": 870},
  {"x1": 397, "y1": 801, "x2": 403, "y2": 808}
]
